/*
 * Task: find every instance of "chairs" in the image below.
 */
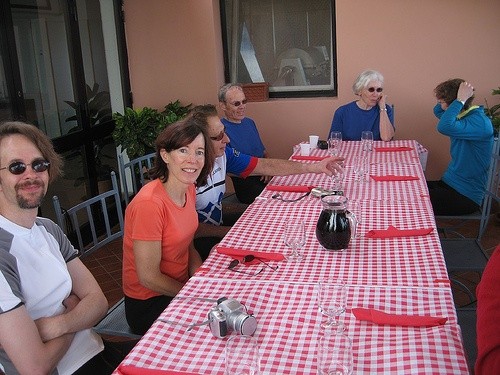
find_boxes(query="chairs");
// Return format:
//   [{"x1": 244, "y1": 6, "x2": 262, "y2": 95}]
[
  {"x1": 53, "y1": 145, "x2": 249, "y2": 347},
  {"x1": 435, "y1": 138, "x2": 500, "y2": 375}
]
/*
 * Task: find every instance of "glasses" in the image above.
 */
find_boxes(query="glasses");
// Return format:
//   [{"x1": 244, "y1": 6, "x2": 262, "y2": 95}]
[
  {"x1": 366, "y1": 87, "x2": 383, "y2": 92},
  {"x1": 437, "y1": 99, "x2": 447, "y2": 104},
  {"x1": 228, "y1": 255, "x2": 279, "y2": 277},
  {"x1": 0, "y1": 159, "x2": 50, "y2": 175},
  {"x1": 224, "y1": 98, "x2": 247, "y2": 106},
  {"x1": 210, "y1": 126, "x2": 226, "y2": 141}
]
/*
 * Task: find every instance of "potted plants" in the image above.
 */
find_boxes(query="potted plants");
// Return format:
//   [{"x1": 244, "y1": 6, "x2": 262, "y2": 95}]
[
  {"x1": 63, "y1": 82, "x2": 112, "y2": 202},
  {"x1": 113, "y1": 100, "x2": 194, "y2": 192}
]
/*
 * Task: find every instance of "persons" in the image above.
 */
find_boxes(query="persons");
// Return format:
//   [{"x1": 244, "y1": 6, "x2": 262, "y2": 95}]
[
  {"x1": 0, "y1": 121, "x2": 127, "y2": 375},
  {"x1": 219, "y1": 83, "x2": 275, "y2": 204},
  {"x1": 474, "y1": 244, "x2": 500, "y2": 375},
  {"x1": 189, "y1": 104, "x2": 346, "y2": 262},
  {"x1": 122, "y1": 120, "x2": 214, "y2": 335},
  {"x1": 427, "y1": 79, "x2": 494, "y2": 216},
  {"x1": 328, "y1": 70, "x2": 395, "y2": 142}
]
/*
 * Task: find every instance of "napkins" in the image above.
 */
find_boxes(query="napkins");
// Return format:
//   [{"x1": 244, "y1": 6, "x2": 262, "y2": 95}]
[
  {"x1": 365, "y1": 225, "x2": 435, "y2": 239},
  {"x1": 370, "y1": 175, "x2": 419, "y2": 180},
  {"x1": 117, "y1": 366, "x2": 200, "y2": 375},
  {"x1": 374, "y1": 147, "x2": 414, "y2": 152},
  {"x1": 217, "y1": 247, "x2": 285, "y2": 266},
  {"x1": 352, "y1": 308, "x2": 446, "y2": 327},
  {"x1": 266, "y1": 184, "x2": 314, "y2": 192},
  {"x1": 292, "y1": 155, "x2": 329, "y2": 161}
]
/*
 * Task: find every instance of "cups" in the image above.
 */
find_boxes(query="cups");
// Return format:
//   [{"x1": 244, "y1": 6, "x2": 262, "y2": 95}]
[
  {"x1": 362, "y1": 131, "x2": 373, "y2": 151},
  {"x1": 224, "y1": 335, "x2": 260, "y2": 375},
  {"x1": 301, "y1": 144, "x2": 309, "y2": 156},
  {"x1": 316, "y1": 332, "x2": 354, "y2": 375},
  {"x1": 309, "y1": 135, "x2": 320, "y2": 148},
  {"x1": 328, "y1": 131, "x2": 342, "y2": 158}
]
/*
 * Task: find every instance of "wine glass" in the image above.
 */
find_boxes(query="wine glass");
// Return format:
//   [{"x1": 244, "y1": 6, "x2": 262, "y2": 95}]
[
  {"x1": 353, "y1": 156, "x2": 368, "y2": 184},
  {"x1": 318, "y1": 278, "x2": 347, "y2": 334},
  {"x1": 283, "y1": 218, "x2": 306, "y2": 262}
]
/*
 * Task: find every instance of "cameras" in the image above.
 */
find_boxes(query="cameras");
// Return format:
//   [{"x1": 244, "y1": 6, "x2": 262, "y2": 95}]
[{"x1": 208, "y1": 297, "x2": 257, "y2": 338}]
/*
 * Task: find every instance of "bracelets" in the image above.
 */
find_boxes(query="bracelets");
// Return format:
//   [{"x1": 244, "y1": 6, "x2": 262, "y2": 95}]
[{"x1": 379, "y1": 108, "x2": 387, "y2": 111}]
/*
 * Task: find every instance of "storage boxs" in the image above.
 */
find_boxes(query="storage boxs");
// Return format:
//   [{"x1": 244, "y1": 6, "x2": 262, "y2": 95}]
[{"x1": 242, "y1": 82, "x2": 271, "y2": 102}]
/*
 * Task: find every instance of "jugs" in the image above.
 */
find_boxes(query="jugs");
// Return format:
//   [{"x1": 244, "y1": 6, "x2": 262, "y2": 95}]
[{"x1": 315, "y1": 195, "x2": 358, "y2": 252}]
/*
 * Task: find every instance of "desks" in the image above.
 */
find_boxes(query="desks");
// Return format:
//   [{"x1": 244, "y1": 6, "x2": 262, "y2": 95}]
[{"x1": 111, "y1": 140, "x2": 471, "y2": 375}]
